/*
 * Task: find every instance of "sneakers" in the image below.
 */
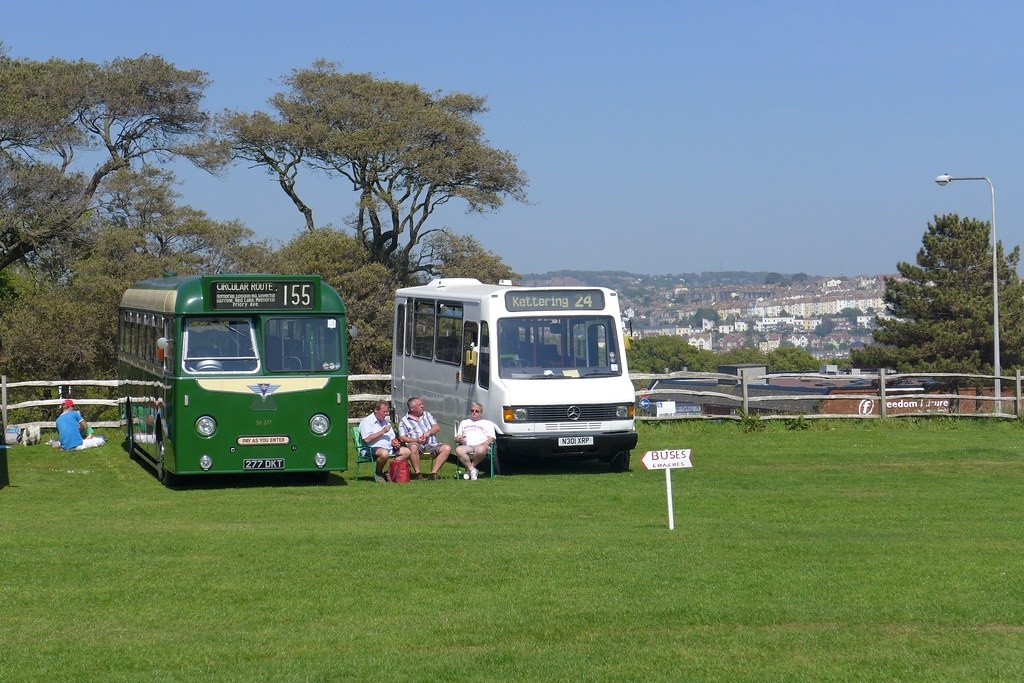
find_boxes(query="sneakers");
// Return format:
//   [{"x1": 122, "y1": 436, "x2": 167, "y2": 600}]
[
  {"x1": 470, "y1": 468, "x2": 479, "y2": 480},
  {"x1": 463, "y1": 467, "x2": 471, "y2": 479}
]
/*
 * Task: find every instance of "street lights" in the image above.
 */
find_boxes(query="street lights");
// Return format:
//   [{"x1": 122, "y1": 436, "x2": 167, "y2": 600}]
[{"x1": 934, "y1": 172, "x2": 1001, "y2": 415}]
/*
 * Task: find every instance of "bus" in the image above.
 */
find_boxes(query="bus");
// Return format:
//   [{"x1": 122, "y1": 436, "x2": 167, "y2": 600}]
[
  {"x1": 389, "y1": 274, "x2": 639, "y2": 474},
  {"x1": 109, "y1": 269, "x2": 359, "y2": 480}
]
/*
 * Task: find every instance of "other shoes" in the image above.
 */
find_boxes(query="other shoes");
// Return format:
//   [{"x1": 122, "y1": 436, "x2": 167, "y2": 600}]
[
  {"x1": 374, "y1": 471, "x2": 387, "y2": 483},
  {"x1": 415, "y1": 473, "x2": 423, "y2": 480},
  {"x1": 428, "y1": 473, "x2": 436, "y2": 481}
]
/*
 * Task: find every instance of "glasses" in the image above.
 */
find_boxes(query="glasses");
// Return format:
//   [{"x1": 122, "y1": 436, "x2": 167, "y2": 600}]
[{"x1": 470, "y1": 409, "x2": 482, "y2": 413}]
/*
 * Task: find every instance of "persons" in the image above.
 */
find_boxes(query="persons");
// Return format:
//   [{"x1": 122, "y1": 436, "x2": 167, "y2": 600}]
[
  {"x1": 454, "y1": 402, "x2": 496, "y2": 480},
  {"x1": 399, "y1": 397, "x2": 451, "y2": 480},
  {"x1": 56, "y1": 400, "x2": 105, "y2": 450},
  {"x1": 358, "y1": 399, "x2": 411, "y2": 482}
]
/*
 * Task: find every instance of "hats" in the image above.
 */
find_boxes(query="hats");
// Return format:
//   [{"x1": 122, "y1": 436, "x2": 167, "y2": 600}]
[{"x1": 62, "y1": 400, "x2": 73, "y2": 407}]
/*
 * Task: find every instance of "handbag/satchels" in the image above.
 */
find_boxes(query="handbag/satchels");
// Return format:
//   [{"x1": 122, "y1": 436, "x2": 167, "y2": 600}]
[{"x1": 389, "y1": 459, "x2": 411, "y2": 483}]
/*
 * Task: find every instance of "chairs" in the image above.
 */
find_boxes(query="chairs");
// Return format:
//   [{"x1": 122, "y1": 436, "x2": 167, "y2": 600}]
[
  {"x1": 350, "y1": 427, "x2": 397, "y2": 483},
  {"x1": 402, "y1": 442, "x2": 436, "y2": 472},
  {"x1": 455, "y1": 440, "x2": 495, "y2": 481}
]
